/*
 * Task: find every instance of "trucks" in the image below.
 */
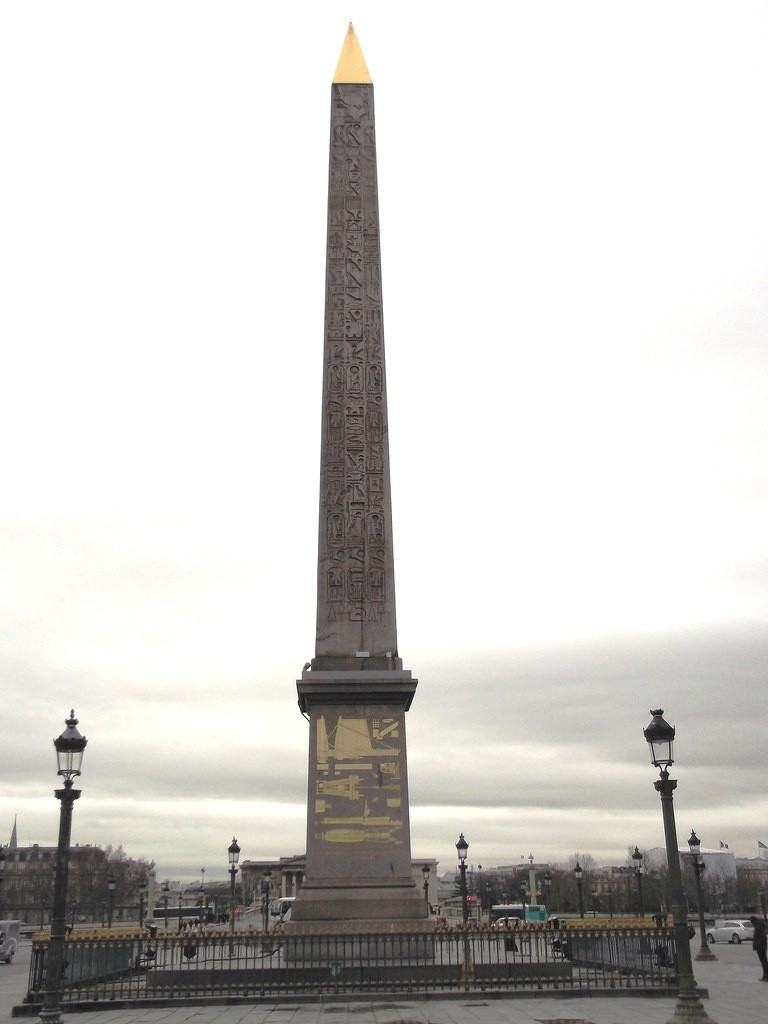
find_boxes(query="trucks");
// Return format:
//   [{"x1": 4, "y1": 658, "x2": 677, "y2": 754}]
[{"x1": 0, "y1": 920, "x2": 21, "y2": 963}]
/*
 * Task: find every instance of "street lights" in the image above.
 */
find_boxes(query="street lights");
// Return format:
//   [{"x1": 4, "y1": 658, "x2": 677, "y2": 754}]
[
  {"x1": 543, "y1": 871, "x2": 551, "y2": 917},
  {"x1": 178, "y1": 893, "x2": 185, "y2": 926},
  {"x1": 101, "y1": 897, "x2": 106, "y2": 928},
  {"x1": 107, "y1": 874, "x2": 119, "y2": 930},
  {"x1": 642, "y1": 707, "x2": 717, "y2": 1024},
  {"x1": 163, "y1": 883, "x2": 171, "y2": 929},
  {"x1": 573, "y1": 861, "x2": 585, "y2": 919},
  {"x1": 38, "y1": 709, "x2": 89, "y2": 1024},
  {"x1": 198, "y1": 885, "x2": 208, "y2": 931},
  {"x1": 262, "y1": 867, "x2": 274, "y2": 954},
  {"x1": 687, "y1": 829, "x2": 718, "y2": 963},
  {"x1": 592, "y1": 888, "x2": 596, "y2": 918},
  {"x1": 225, "y1": 837, "x2": 241, "y2": 957},
  {"x1": 70, "y1": 896, "x2": 77, "y2": 931},
  {"x1": 632, "y1": 883, "x2": 640, "y2": 918},
  {"x1": 520, "y1": 880, "x2": 526, "y2": 918},
  {"x1": 630, "y1": 846, "x2": 646, "y2": 919},
  {"x1": 607, "y1": 888, "x2": 613, "y2": 919},
  {"x1": 455, "y1": 832, "x2": 478, "y2": 978},
  {"x1": 137, "y1": 879, "x2": 146, "y2": 930},
  {"x1": 420, "y1": 863, "x2": 431, "y2": 917}
]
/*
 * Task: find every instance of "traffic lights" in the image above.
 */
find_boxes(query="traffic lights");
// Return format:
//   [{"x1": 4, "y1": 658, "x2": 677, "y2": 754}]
[
  {"x1": 468, "y1": 896, "x2": 472, "y2": 902},
  {"x1": 236, "y1": 905, "x2": 241, "y2": 913}
]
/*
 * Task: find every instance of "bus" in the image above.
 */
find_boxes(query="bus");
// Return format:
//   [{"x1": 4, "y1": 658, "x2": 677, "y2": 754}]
[
  {"x1": 271, "y1": 897, "x2": 299, "y2": 935},
  {"x1": 490, "y1": 904, "x2": 548, "y2": 922}
]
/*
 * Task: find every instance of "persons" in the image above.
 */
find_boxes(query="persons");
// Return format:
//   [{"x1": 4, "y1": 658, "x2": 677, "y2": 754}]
[
  {"x1": 178, "y1": 918, "x2": 198, "y2": 932},
  {"x1": 749, "y1": 915, "x2": 768, "y2": 982},
  {"x1": 223, "y1": 913, "x2": 229, "y2": 924}
]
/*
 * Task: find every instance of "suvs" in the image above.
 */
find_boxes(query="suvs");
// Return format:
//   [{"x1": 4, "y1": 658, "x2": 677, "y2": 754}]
[{"x1": 490, "y1": 917, "x2": 524, "y2": 929}]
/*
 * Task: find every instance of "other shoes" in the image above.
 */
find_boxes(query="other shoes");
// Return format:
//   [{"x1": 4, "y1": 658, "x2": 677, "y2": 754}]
[{"x1": 759, "y1": 977, "x2": 768, "y2": 982}]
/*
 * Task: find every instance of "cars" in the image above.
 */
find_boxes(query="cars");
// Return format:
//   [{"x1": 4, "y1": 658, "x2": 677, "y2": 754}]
[
  {"x1": 435, "y1": 917, "x2": 448, "y2": 927},
  {"x1": 706, "y1": 920, "x2": 756, "y2": 947},
  {"x1": 467, "y1": 917, "x2": 481, "y2": 929}
]
